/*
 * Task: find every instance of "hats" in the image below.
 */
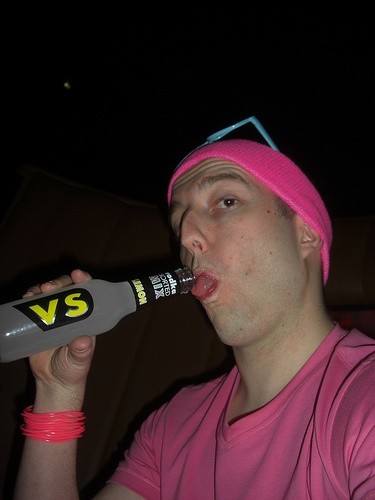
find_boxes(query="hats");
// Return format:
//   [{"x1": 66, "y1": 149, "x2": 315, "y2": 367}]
[{"x1": 168, "y1": 138, "x2": 331, "y2": 286}]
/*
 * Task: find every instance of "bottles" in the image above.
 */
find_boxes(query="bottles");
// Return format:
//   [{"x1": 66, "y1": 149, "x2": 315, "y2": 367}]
[{"x1": 1, "y1": 264, "x2": 194, "y2": 361}]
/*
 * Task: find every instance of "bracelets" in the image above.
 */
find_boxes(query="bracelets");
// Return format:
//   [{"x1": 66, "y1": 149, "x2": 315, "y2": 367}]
[{"x1": 18, "y1": 405, "x2": 88, "y2": 443}]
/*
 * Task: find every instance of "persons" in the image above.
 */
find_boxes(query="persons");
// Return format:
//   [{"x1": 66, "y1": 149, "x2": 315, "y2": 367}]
[{"x1": 12, "y1": 127, "x2": 375, "y2": 498}]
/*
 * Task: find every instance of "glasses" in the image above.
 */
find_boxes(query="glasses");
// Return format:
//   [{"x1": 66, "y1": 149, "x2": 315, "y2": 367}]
[{"x1": 172, "y1": 115, "x2": 282, "y2": 169}]
